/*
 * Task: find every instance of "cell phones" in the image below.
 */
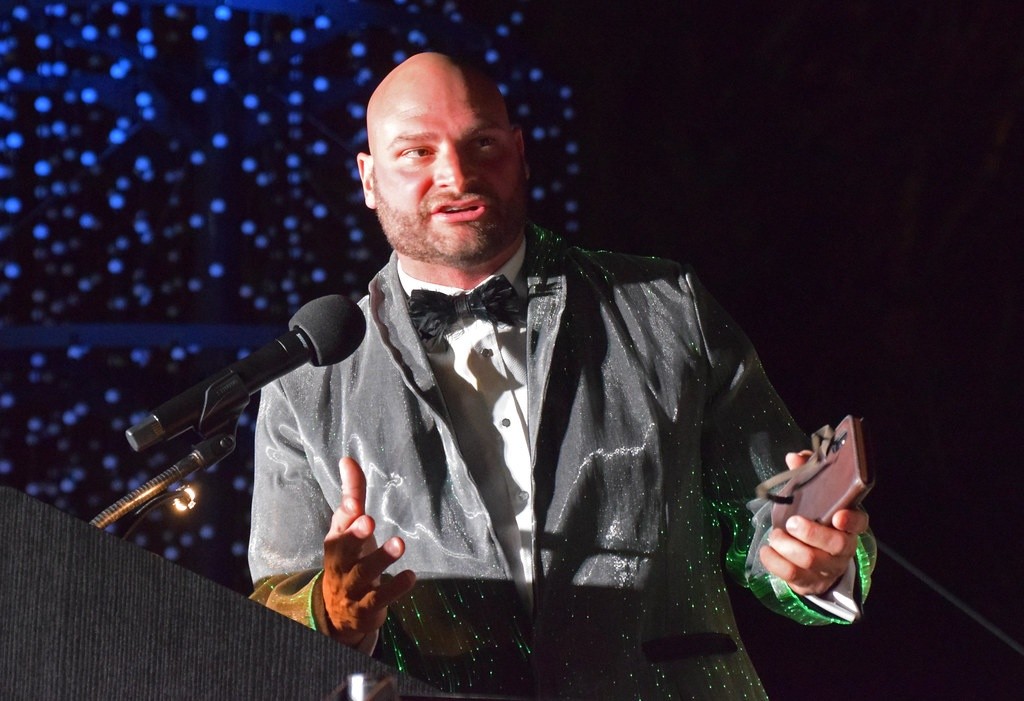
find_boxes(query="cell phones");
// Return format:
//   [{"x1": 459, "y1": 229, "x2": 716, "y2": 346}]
[{"x1": 772, "y1": 411, "x2": 876, "y2": 536}]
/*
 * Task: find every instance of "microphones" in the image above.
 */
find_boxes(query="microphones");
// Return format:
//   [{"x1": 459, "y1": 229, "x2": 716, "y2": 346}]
[{"x1": 125, "y1": 294, "x2": 366, "y2": 452}]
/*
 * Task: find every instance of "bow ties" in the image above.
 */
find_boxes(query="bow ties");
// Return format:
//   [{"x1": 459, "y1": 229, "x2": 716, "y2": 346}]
[{"x1": 410, "y1": 273, "x2": 524, "y2": 347}]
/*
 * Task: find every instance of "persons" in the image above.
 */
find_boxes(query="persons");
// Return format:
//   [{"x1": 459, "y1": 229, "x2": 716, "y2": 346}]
[{"x1": 246, "y1": 52, "x2": 878, "y2": 701}]
